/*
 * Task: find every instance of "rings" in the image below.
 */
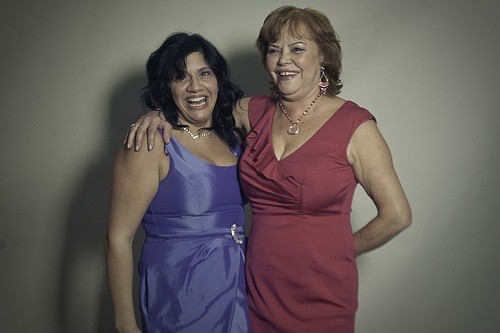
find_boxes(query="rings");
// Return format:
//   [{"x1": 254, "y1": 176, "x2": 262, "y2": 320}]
[{"x1": 131, "y1": 124, "x2": 139, "y2": 128}]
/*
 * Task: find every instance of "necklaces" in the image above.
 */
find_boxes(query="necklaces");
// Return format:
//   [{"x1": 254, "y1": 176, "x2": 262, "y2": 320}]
[
  {"x1": 278, "y1": 90, "x2": 324, "y2": 135},
  {"x1": 176, "y1": 121, "x2": 213, "y2": 140}
]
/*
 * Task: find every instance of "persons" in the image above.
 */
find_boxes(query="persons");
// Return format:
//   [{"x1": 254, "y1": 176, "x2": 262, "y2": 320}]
[
  {"x1": 105, "y1": 31, "x2": 250, "y2": 333},
  {"x1": 124, "y1": 6, "x2": 412, "y2": 333}
]
